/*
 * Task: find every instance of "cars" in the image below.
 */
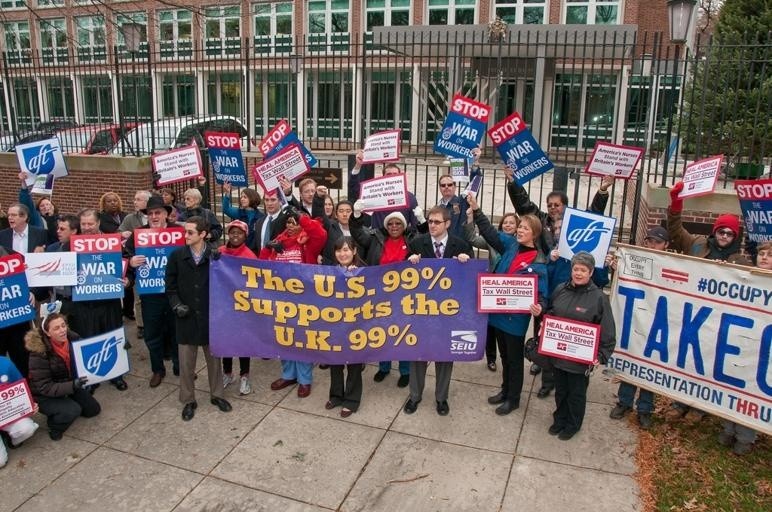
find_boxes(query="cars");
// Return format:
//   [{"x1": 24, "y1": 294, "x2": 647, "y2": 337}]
[
  {"x1": 1, "y1": 129, "x2": 53, "y2": 151},
  {"x1": 47, "y1": 121, "x2": 148, "y2": 156}
]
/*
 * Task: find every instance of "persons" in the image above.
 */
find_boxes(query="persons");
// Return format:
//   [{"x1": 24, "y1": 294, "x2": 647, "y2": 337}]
[
  {"x1": 221, "y1": 148, "x2": 481, "y2": 417},
  {"x1": 1, "y1": 172, "x2": 233, "y2": 467},
  {"x1": 466, "y1": 167, "x2": 618, "y2": 440},
  {"x1": 610, "y1": 182, "x2": 771, "y2": 458}
]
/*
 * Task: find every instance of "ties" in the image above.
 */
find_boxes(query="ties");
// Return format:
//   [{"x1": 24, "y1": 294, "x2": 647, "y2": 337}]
[
  {"x1": 264, "y1": 215, "x2": 273, "y2": 246},
  {"x1": 434, "y1": 242, "x2": 443, "y2": 258}
]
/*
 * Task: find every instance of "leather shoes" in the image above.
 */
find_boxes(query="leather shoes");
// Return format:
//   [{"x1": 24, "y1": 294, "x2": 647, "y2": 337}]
[
  {"x1": 211, "y1": 397, "x2": 232, "y2": 412},
  {"x1": 488, "y1": 360, "x2": 497, "y2": 371},
  {"x1": 115, "y1": 379, "x2": 127, "y2": 391},
  {"x1": 174, "y1": 369, "x2": 197, "y2": 380},
  {"x1": 49, "y1": 429, "x2": 62, "y2": 441},
  {"x1": 127, "y1": 314, "x2": 135, "y2": 321},
  {"x1": 530, "y1": 363, "x2": 577, "y2": 440},
  {"x1": 137, "y1": 328, "x2": 144, "y2": 339},
  {"x1": 488, "y1": 392, "x2": 506, "y2": 405},
  {"x1": 271, "y1": 379, "x2": 352, "y2": 418},
  {"x1": 374, "y1": 370, "x2": 449, "y2": 416},
  {"x1": 183, "y1": 402, "x2": 197, "y2": 420},
  {"x1": 610, "y1": 404, "x2": 754, "y2": 455},
  {"x1": 150, "y1": 373, "x2": 161, "y2": 387},
  {"x1": 495, "y1": 398, "x2": 519, "y2": 416}
]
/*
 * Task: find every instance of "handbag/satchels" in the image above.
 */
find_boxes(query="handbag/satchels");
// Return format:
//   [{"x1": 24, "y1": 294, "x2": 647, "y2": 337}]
[{"x1": 524, "y1": 336, "x2": 549, "y2": 367}]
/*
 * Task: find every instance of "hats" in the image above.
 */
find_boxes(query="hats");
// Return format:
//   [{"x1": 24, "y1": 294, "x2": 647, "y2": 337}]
[
  {"x1": 139, "y1": 196, "x2": 172, "y2": 216},
  {"x1": 644, "y1": 227, "x2": 670, "y2": 243},
  {"x1": 571, "y1": 251, "x2": 595, "y2": 269},
  {"x1": 227, "y1": 219, "x2": 248, "y2": 232},
  {"x1": 384, "y1": 212, "x2": 407, "y2": 230},
  {"x1": 713, "y1": 214, "x2": 740, "y2": 240}
]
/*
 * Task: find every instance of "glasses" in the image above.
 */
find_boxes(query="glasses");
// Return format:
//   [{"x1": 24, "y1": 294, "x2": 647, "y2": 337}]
[
  {"x1": 584, "y1": 364, "x2": 593, "y2": 377},
  {"x1": 427, "y1": 219, "x2": 446, "y2": 225},
  {"x1": 440, "y1": 183, "x2": 453, "y2": 187},
  {"x1": 719, "y1": 229, "x2": 735, "y2": 237}
]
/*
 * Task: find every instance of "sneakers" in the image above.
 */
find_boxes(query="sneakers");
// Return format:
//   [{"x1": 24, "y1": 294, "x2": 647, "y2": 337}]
[
  {"x1": 239, "y1": 376, "x2": 250, "y2": 395},
  {"x1": 222, "y1": 373, "x2": 236, "y2": 389}
]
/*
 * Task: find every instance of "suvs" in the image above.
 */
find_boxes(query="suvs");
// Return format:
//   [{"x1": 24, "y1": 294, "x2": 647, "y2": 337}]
[{"x1": 106, "y1": 113, "x2": 253, "y2": 157}]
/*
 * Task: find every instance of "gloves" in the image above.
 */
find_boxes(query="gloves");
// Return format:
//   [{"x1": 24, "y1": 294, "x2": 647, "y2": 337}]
[
  {"x1": 412, "y1": 206, "x2": 426, "y2": 224},
  {"x1": 283, "y1": 205, "x2": 298, "y2": 217},
  {"x1": 670, "y1": 182, "x2": 684, "y2": 213},
  {"x1": 354, "y1": 199, "x2": 364, "y2": 218},
  {"x1": 75, "y1": 376, "x2": 88, "y2": 389},
  {"x1": 173, "y1": 302, "x2": 189, "y2": 318},
  {"x1": 124, "y1": 342, "x2": 131, "y2": 349},
  {"x1": 210, "y1": 248, "x2": 220, "y2": 260}
]
